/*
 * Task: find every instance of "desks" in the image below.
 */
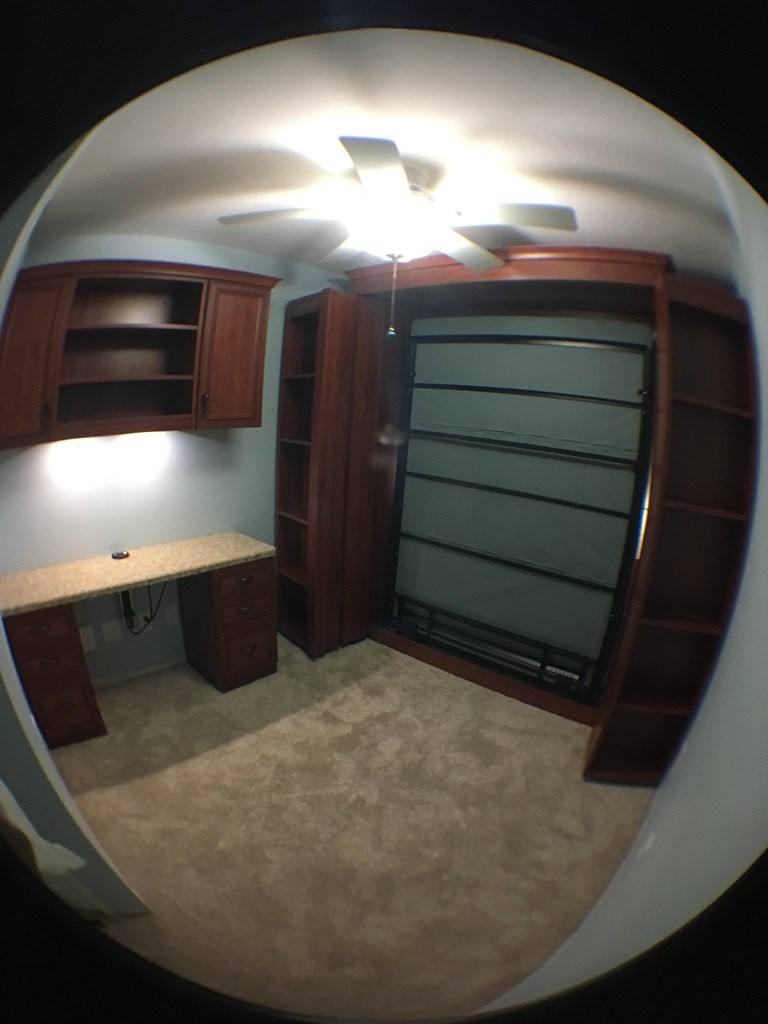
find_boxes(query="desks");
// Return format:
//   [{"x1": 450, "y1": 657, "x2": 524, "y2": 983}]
[{"x1": 1, "y1": 531, "x2": 278, "y2": 750}]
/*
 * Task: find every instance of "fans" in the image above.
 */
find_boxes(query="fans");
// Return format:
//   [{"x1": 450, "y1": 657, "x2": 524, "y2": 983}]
[{"x1": 216, "y1": 135, "x2": 578, "y2": 341}]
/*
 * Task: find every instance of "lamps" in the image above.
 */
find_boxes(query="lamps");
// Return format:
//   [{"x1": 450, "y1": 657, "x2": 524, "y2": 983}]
[{"x1": 341, "y1": 183, "x2": 446, "y2": 263}]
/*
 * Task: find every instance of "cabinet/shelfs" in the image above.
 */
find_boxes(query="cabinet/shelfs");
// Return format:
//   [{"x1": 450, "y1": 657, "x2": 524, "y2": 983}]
[
  {"x1": 579, "y1": 274, "x2": 760, "y2": 789},
  {"x1": 1, "y1": 258, "x2": 283, "y2": 452},
  {"x1": 273, "y1": 289, "x2": 389, "y2": 662}
]
